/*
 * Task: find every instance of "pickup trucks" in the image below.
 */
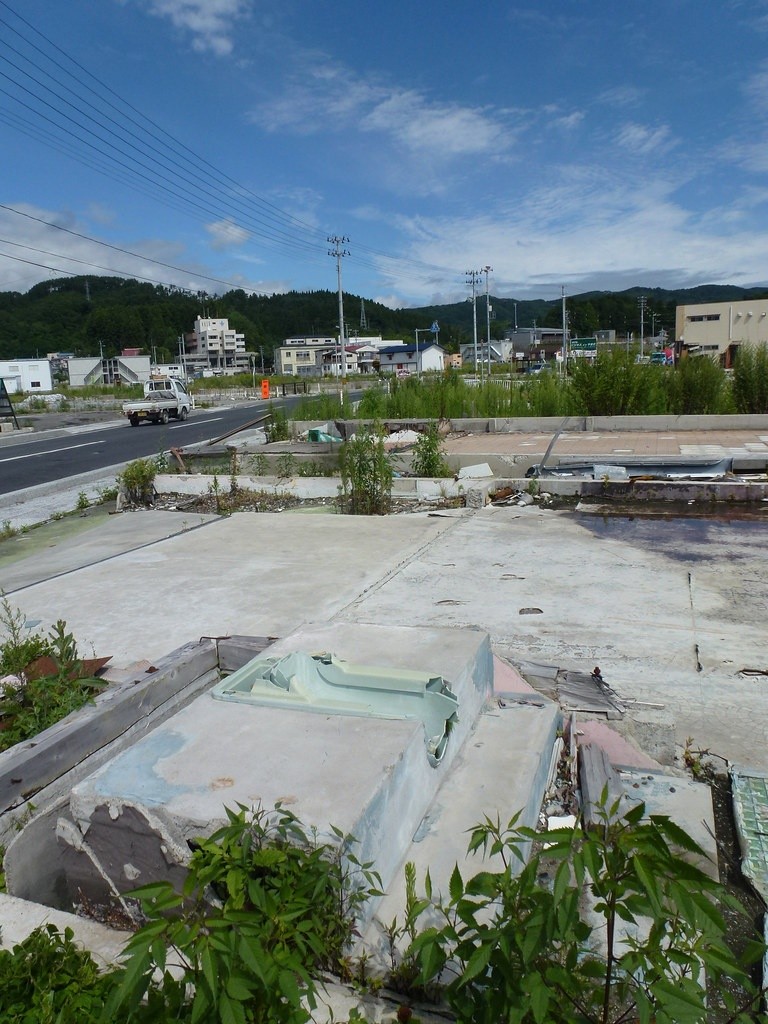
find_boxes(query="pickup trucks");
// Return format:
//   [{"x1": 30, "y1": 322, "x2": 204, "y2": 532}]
[{"x1": 123, "y1": 378, "x2": 194, "y2": 426}]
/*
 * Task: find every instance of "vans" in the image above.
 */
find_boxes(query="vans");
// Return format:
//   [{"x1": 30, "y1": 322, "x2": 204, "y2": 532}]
[{"x1": 529, "y1": 363, "x2": 552, "y2": 374}]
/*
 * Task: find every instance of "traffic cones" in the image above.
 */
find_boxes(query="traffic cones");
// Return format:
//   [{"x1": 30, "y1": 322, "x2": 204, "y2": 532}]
[{"x1": 308, "y1": 429, "x2": 343, "y2": 443}]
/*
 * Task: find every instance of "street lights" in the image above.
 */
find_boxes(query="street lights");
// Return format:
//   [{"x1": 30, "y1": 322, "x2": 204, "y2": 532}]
[{"x1": 415, "y1": 327, "x2": 440, "y2": 379}]
